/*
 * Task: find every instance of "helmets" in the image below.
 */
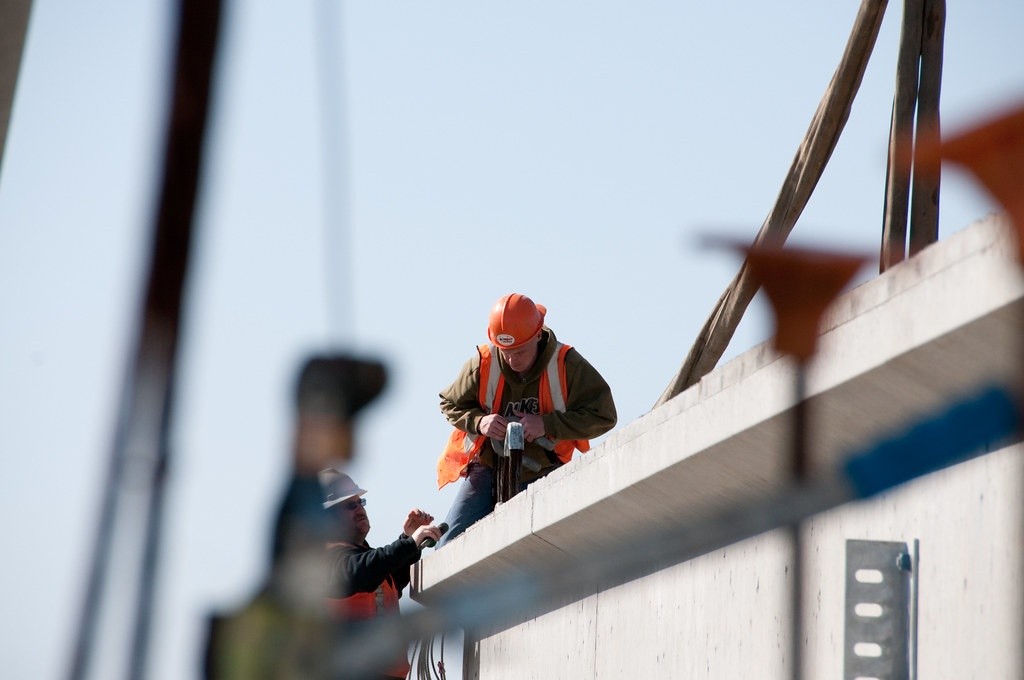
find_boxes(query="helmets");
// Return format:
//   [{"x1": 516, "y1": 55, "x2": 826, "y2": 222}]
[
  {"x1": 317, "y1": 468, "x2": 367, "y2": 509},
  {"x1": 487, "y1": 293, "x2": 547, "y2": 349}
]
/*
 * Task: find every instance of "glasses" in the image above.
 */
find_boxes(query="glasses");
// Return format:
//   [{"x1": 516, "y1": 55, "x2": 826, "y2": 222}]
[{"x1": 333, "y1": 499, "x2": 367, "y2": 515}]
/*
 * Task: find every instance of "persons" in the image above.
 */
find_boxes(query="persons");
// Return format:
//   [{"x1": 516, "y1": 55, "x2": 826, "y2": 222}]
[
  {"x1": 317, "y1": 468, "x2": 443, "y2": 680},
  {"x1": 435, "y1": 292, "x2": 617, "y2": 551}
]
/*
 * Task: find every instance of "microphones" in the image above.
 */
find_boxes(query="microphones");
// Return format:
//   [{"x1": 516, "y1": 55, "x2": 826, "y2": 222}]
[{"x1": 419, "y1": 523, "x2": 449, "y2": 550}]
